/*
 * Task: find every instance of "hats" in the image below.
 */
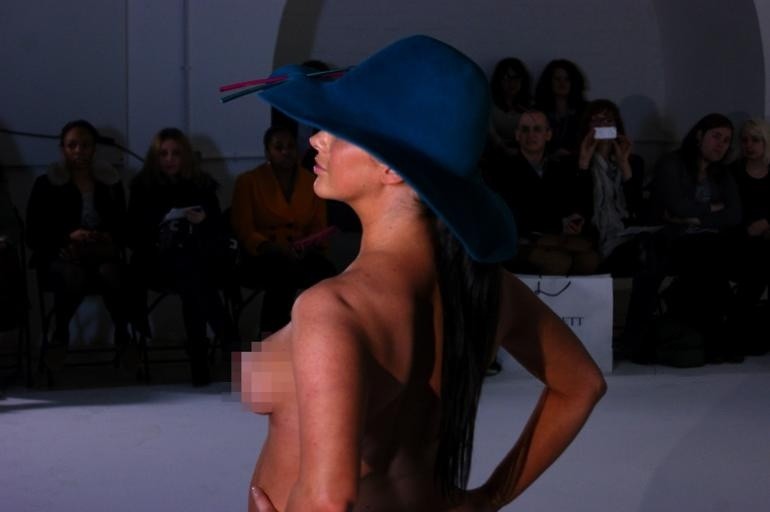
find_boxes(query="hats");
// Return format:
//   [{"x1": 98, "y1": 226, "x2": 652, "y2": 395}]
[{"x1": 215, "y1": 33, "x2": 530, "y2": 271}]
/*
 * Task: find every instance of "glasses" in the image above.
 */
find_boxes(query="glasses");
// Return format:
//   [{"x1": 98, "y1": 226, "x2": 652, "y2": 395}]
[{"x1": 591, "y1": 117, "x2": 617, "y2": 128}]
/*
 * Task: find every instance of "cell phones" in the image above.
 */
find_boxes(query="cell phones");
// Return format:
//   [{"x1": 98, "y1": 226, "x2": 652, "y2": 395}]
[
  {"x1": 571, "y1": 217, "x2": 583, "y2": 226},
  {"x1": 594, "y1": 127, "x2": 617, "y2": 140}
]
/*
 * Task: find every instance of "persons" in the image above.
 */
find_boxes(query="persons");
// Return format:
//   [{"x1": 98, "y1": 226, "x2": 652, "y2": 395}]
[
  {"x1": 220, "y1": 33, "x2": 607, "y2": 511},
  {"x1": 470, "y1": 56, "x2": 770, "y2": 368},
  {"x1": 25, "y1": 120, "x2": 134, "y2": 370},
  {"x1": 122, "y1": 125, "x2": 224, "y2": 389},
  {"x1": 230, "y1": 121, "x2": 332, "y2": 342}
]
